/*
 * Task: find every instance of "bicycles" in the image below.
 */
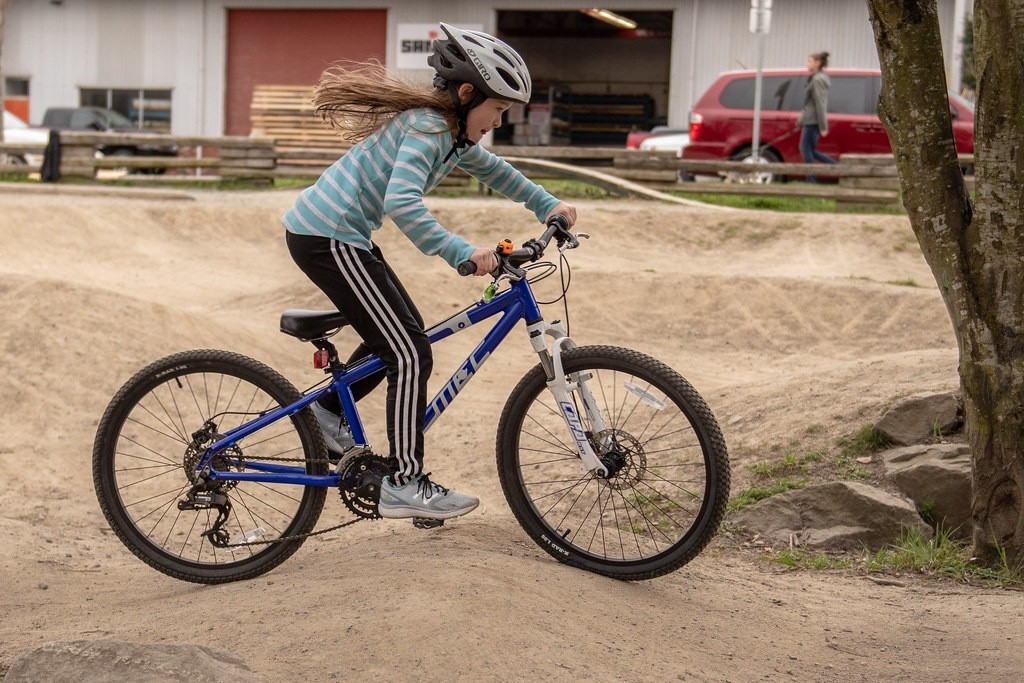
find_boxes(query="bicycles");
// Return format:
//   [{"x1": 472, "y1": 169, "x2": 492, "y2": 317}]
[{"x1": 90, "y1": 213, "x2": 730, "y2": 584}]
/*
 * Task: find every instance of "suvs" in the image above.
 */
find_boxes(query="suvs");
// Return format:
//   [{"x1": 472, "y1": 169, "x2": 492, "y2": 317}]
[{"x1": 37, "y1": 104, "x2": 176, "y2": 173}]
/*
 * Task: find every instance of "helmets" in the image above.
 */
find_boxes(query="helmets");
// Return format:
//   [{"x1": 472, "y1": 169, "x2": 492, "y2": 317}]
[{"x1": 429, "y1": 23, "x2": 533, "y2": 106}]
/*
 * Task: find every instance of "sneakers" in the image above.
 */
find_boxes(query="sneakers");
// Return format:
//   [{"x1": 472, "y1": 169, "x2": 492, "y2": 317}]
[
  {"x1": 309, "y1": 400, "x2": 356, "y2": 456},
  {"x1": 378, "y1": 476, "x2": 480, "y2": 519}
]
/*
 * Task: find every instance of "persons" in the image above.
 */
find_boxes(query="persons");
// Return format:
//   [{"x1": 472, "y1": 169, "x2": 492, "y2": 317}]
[
  {"x1": 793, "y1": 50, "x2": 840, "y2": 183},
  {"x1": 960, "y1": 75, "x2": 977, "y2": 104},
  {"x1": 283, "y1": 41, "x2": 575, "y2": 520}
]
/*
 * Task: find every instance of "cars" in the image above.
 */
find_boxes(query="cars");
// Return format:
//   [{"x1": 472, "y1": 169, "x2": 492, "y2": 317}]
[{"x1": 678, "y1": 62, "x2": 976, "y2": 187}]
